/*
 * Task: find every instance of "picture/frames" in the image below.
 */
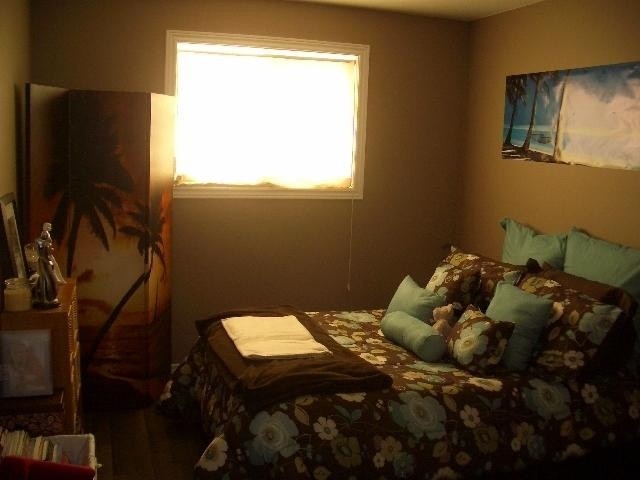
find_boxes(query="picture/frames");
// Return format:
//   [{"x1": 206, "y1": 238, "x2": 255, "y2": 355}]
[{"x1": 0, "y1": 189, "x2": 29, "y2": 279}]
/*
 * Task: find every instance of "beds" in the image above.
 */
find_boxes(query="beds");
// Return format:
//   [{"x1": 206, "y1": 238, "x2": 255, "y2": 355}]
[{"x1": 155, "y1": 309, "x2": 638, "y2": 480}]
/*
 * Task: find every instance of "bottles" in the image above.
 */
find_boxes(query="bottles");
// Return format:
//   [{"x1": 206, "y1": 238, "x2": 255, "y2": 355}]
[{"x1": 4, "y1": 277, "x2": 32, "y2": 313}]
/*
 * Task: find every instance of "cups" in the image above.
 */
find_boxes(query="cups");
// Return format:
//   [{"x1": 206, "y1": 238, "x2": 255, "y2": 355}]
[{"x1": 24, "y1": 243, "x2": 38, "y2": 270}]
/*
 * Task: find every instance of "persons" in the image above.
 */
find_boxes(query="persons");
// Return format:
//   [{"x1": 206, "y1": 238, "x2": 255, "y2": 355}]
[{"x1": 33, "y1": 222, "x2": 67, "y2": 309}]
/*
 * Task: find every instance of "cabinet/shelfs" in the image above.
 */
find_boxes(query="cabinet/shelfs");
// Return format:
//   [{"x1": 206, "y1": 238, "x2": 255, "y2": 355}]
[{"x1": 0, "y1": 278, "x2": 81, "y2": 432}]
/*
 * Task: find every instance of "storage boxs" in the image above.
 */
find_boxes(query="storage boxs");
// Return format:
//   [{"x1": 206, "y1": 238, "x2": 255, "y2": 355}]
[{"x1": 0, "y1": 433, "x2": 97, "y2": 480}]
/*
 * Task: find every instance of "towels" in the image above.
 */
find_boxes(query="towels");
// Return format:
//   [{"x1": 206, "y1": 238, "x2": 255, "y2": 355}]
[{"x1": 219, "y1": 314, "x2": 332, "y2": 361}]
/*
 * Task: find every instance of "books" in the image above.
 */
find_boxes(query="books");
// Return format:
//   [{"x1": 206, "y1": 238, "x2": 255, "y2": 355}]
[{"x1": 1, "y1": 430, "x2": 70, "y2": 464}]
